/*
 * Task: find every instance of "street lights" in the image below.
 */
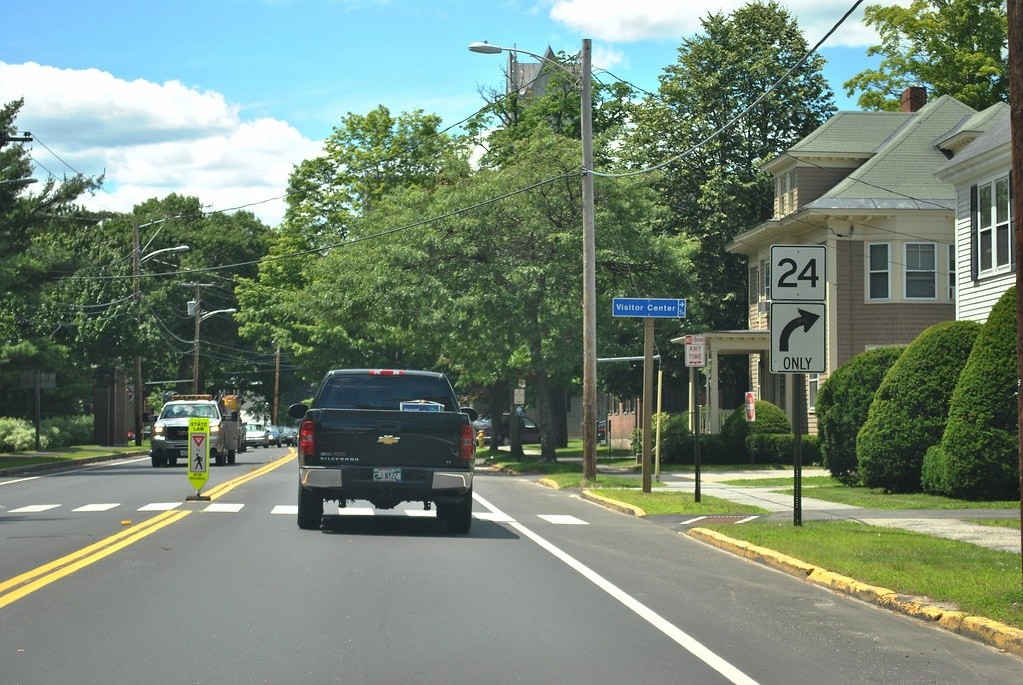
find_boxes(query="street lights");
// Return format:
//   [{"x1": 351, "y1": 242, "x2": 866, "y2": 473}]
[
  {"x1": 130, "y1": 245, "x2": 190, "y2": 446},
  {"x1": 192, "y1": 305, "x2": 236, "y2": 394},
  {"x1": 467, "y1": 37, "x2": 598, "y2": 483}
]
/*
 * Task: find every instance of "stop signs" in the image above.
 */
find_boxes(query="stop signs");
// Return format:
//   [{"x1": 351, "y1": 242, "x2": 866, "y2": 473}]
[{"x1": 745, "y1": 393, "x2": 755, "y2": 420}]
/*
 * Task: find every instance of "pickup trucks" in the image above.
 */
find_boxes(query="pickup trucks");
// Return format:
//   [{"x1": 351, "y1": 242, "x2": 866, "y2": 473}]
[
  {"x1": 150, "y1": 393, "x2": 239, "y2": 466},
  {"x1": 288, "y1": 368, "x2": 477, "y2": 536}
]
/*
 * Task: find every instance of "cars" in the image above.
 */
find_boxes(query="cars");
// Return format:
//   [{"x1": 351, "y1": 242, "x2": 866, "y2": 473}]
[
  {"x1": 239, "y1": 418, "x2": 248, "y2": 452},
  {"x1": 246, "y1": 424, "x2": 268, "y2": 448},
  {"x1": 282, "y1": 426, "x2": 298, "y2": 445},
  {"x1": 598, "y1": 421, "x2": 606, "y2": 442},
  {"x1": 474, "y1": 413, "x2": 542, "y2": 446},
  {"x1": 266, "y1": 426, "x2": 280, "y2": 447}
]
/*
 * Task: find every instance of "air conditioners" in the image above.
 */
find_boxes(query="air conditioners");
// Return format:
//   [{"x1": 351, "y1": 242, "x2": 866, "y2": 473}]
[{"x1": 757, "y1": 301, "x2": 770, "y2": 313}]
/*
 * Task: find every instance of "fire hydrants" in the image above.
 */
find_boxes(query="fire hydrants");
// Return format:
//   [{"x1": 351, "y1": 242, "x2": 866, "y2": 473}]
[{"x1": 478, "y1": 430, "x2": 484, "y2": 447}]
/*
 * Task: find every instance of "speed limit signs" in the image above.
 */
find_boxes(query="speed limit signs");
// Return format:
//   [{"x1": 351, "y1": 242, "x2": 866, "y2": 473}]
[{"x1": 769, "y1": 245, "x2": 826, "y2": 300}]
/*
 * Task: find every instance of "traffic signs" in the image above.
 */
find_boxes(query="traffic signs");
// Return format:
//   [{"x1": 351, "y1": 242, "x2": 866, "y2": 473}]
[
  {"x1": 612, "y1": 298, "x2": 687, "y2": 318},
  {"x1": 685, "y1": 335, "x2": 706, "y2": 366}
]
